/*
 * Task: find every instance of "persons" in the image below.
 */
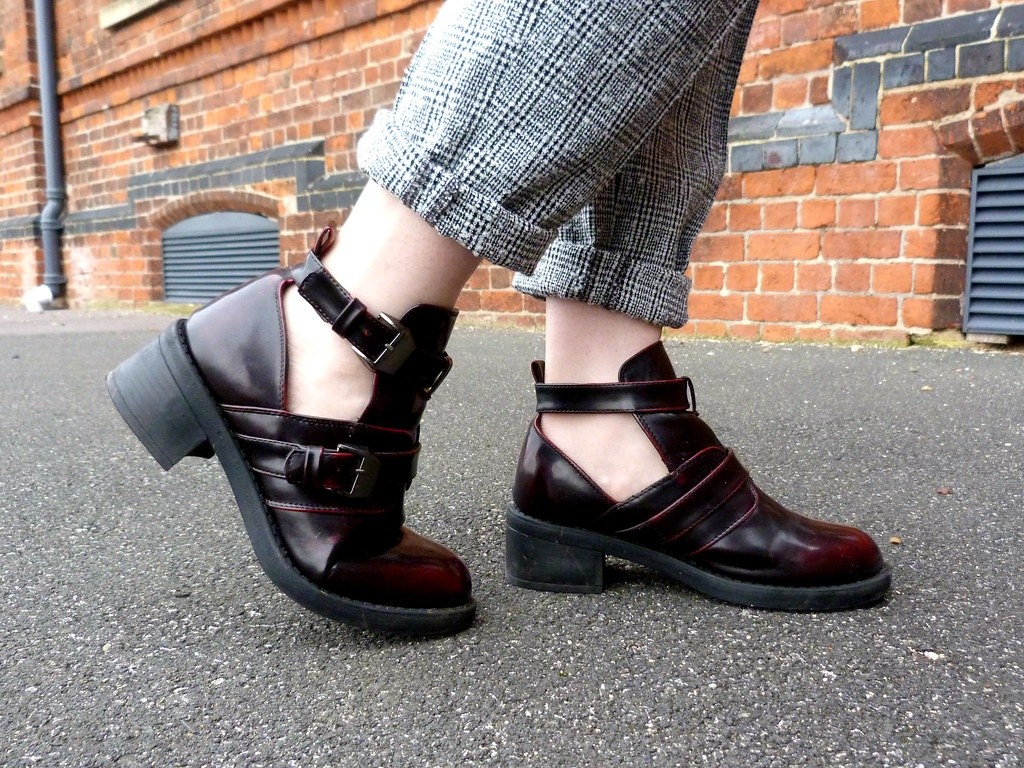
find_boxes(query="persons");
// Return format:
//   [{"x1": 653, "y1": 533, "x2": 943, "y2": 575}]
[{"x1": 103, "y1": 0, "x2": 892, "y2": 631}]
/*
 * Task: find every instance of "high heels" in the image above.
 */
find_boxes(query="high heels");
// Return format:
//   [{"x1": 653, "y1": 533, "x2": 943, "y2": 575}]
[
  {"x1": 499, "y1": 334, "x2": 896, "y2": 618},
  {"x1": 103, "y1": 225, "x2": 481, "y2": 642}
]
